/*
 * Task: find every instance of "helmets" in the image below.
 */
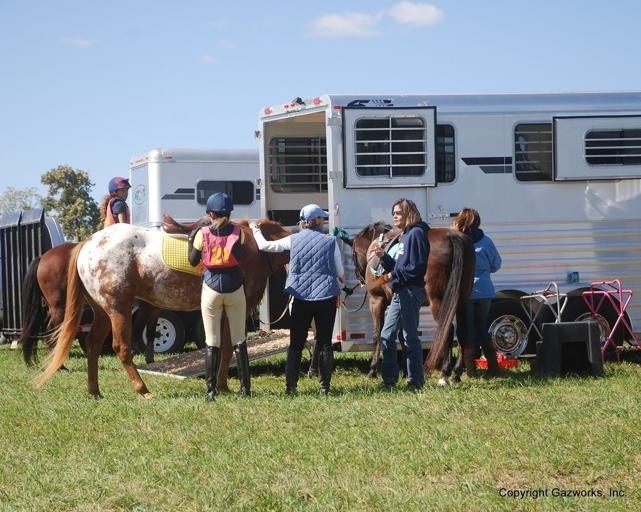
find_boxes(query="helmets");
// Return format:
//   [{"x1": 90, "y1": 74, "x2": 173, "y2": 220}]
[
  {"x1": 207, "y1": 192, "x2": 233, "y2": 212},
  {"x1": 108, "y1": 176, "x2": 131, "y2": 192}
]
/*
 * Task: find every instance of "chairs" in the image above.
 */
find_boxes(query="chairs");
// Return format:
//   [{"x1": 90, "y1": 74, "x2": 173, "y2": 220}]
[{"x1": 535, "y1": 321, "x2": 602, "y2": 377}]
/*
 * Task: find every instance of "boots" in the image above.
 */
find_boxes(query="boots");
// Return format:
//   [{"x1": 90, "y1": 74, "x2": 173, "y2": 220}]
[
  {"x1": 234, "y1": 342, "x2": 251, "y2": 397},
  {"x1": 205, "y1": 346, "x2": 220, "y2": 401}
]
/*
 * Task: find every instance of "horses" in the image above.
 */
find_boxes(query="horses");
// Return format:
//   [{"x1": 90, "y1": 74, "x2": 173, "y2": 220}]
[
  {"x1": 19, "y1": 238, "x2": 161, "y2": 371},
  {"x1": 32, "y1": 218, "x2": 291, "y2": 401},
  {"x1": 337, "y1": 219, "x2": 477, "y2": 389}
]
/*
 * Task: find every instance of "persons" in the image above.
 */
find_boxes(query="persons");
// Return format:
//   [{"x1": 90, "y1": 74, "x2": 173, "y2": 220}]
[
  {"x1": 367, "y1": 198, "x2": 432, "y2": 394},
  {"x1": 100, "y1": 176, "x2": 133, "y2": 230},
  {"x1": 248, "y1": 203, "x2": 349, "y2": 397},
  {"x1": 188, "y1": 191, "x2": 253, "y2": 402},
  {"x1": 452, "y1": 208, "x2": 506, "y2": 380}
]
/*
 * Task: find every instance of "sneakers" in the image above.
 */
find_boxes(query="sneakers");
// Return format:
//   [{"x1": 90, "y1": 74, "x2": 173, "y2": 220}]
[
  {"x1": 286, "y1": 384, "x2": 296, "y2": 394},
  {"x1": 317, "y1": 387, "x2": 328, "y2": 394}
]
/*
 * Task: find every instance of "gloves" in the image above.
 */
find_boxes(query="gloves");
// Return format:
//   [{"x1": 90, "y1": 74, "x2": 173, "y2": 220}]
[{"x1": 189, "y1": 227, "x2": 201, "y2": 239}]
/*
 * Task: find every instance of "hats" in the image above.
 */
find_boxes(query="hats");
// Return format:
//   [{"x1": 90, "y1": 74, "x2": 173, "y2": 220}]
[{"x1": 300, "y1": 204, "x2": 331, "y2": 222}]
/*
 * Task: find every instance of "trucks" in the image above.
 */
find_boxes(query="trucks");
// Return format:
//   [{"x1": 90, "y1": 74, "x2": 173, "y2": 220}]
[
  {"x1": 130, "y1": 147, "x2": 260, "y2": 355},
  {"x1": 138, "y1": 96, "x2": 639, "y2": 401}
]
